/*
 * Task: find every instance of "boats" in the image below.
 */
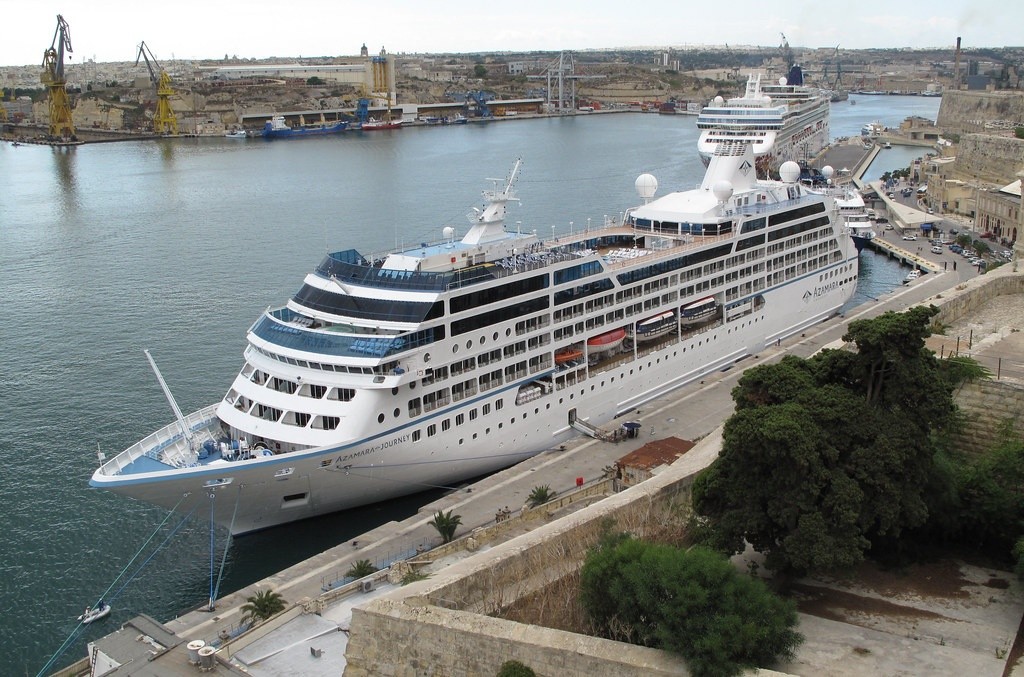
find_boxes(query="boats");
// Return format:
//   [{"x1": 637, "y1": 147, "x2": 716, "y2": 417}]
[
  {"x1": 261, "y1": 97, "x2": 467, "y2": 141},
  {"x1": 815, "y1": 183, "x2": 876, "y2": 252},
  {"x1": 634, "y1": 310, "x2": 677, "y2": 343},
  {"x1": 226, "y1": 131, "x2": 247, "y2": 139},
  {"x1": 555, "y1": 349, "x2": 583, "y2": 364},
  {"x1": 586, "y1": 329, "x2": 627, "y2": 355},
  {"x1": 77, "y1": 605, "x2": 110, "y2": 623},
  {"x1": 903, "y1": 269, "x2": 920, "y2": 283},
  {"x1": 680, "y1": 298, "x2": 718, "y2": 325}
]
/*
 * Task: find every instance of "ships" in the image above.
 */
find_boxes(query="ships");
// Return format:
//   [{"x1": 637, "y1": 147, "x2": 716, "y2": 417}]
[
  {"x1": 84, "y1": 133, "x2": 860, "y2": 536},
  {"x1": 694, "y1": 72, "x2": 833, "y2": 178}
]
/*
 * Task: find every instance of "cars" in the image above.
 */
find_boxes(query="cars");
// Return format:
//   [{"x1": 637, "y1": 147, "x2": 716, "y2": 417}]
[
  {"x1": 876, "y1": 218, "x2": 888, "y2": 223},
  {"x1": 886, "y1": 225, "x2": 894, "y2": 231},
  {"x1": 930, "y1": 238, "x2": 1014, "y2": 266},
  {"x1": 902, "y1": 235, "x2": 916, "y2": 241},
  {"x1": 885, "y1": 185, "x2": 914, "y2": 200}
]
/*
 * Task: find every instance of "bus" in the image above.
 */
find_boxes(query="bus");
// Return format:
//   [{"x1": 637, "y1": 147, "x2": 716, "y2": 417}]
[{"x1": 917, "y1": 184, "x2": 928, "y2": 198}]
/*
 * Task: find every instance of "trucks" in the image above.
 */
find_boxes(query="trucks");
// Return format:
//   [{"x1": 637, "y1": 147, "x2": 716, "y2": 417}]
[{"x1": 865, "y1": 208, "x2": 876, "y2": 220}]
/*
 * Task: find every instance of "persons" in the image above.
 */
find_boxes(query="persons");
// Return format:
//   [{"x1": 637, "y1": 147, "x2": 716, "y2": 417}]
[
  {"x1": 953, "y1": 261, "x2": 956, "y2": 270},
  {"x1": 97, "y1": 597, "x2": 104, "y2": 612}
]
